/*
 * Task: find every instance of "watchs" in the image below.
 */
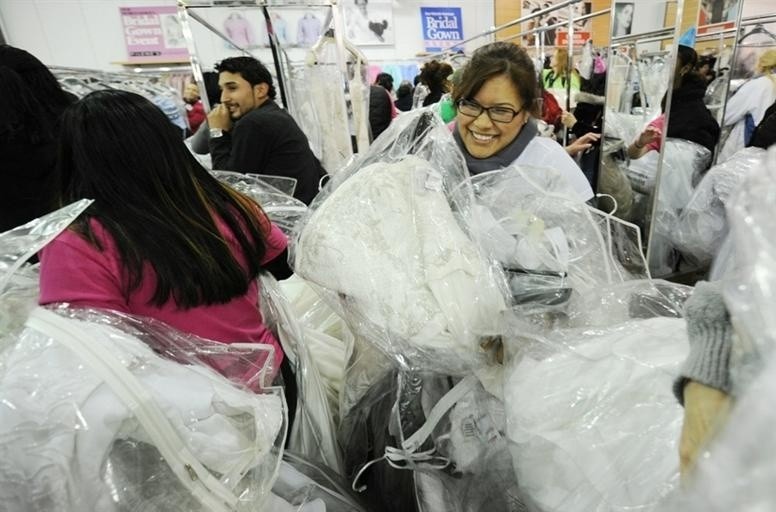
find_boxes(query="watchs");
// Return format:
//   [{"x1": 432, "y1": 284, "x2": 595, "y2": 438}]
[{"x1": 207, "y1": 125, "x2": 227, "y2": 140}]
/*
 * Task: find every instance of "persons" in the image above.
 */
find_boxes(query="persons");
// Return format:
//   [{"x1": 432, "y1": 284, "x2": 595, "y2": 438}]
[
  {"x1": 33, "y1": 86, "x2": 300, "y2": 456},
  {"x1": 190, "y1": 55, "x2": 330, "y2": 208},
  {"x1": 445, "y1": 42, "x2": 599, "y2": 211},
  {"x1": 180, "y1": 84, "x2": 207, "y2": 134},
  {"x1": 0, "y1": 44, "x2": 68, "y2": 238},
  {"x1": 614, "y1": 2, "x2": 634, "y2": 37},
  {"x1": 669, "y1": 273, "x2": 757, "y2": 494},
  {"x1": 367, "y1": 43, "x2": 776, "y2": 167}
]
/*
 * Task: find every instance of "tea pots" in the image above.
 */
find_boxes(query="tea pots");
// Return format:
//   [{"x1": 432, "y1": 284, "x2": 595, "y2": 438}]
[{"x1": 599, "y1": 33, "x2": 679, "y2": 277}]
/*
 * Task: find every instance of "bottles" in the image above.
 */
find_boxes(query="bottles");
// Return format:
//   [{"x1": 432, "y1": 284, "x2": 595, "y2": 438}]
[{"x1": 184, "y1": 84, "x2": 200, "y2": 100}]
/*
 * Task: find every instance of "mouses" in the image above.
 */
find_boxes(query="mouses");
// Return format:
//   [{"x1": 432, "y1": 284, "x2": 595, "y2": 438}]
[
  {"x1": 306, "y1": 13, "x2": 367, "y2": 67},
  {"x1": 737, "y1": 15, "x2": 776, "y2": 44},
  {"x1": 647, "y1": 52, "x2": 666, "y2": 69},
  {"x1": 612, "y1": 45, "x2": 632, "y2": 63}
]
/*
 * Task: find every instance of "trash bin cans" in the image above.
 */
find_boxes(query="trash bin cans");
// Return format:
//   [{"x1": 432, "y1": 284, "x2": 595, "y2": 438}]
[{"x1": 207, "y1": 125, "x2": 227, "y2": 140}]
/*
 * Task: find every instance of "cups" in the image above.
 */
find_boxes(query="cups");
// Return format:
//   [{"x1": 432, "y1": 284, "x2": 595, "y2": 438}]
[{"x1": 457, "y1": 99, "x2": 526, "y2": 124}]
